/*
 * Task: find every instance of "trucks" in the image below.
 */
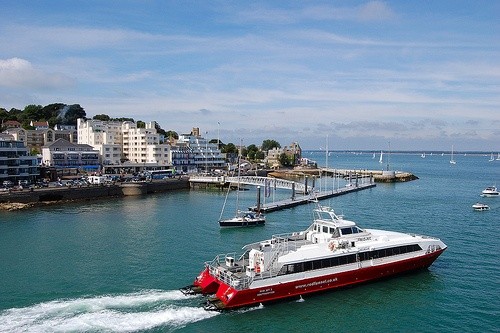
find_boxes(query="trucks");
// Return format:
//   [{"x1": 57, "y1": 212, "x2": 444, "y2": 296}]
[
  {"x1": 87, "y1": 176, "x2": 104, "y2": 184},
  {"x1": 211, "y1": 169, "x2": 226, "y2": 175}
]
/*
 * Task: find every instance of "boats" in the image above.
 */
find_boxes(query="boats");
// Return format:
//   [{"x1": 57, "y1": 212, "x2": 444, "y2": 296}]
[
  {"x1": 472, "y1": 202, "x2": 490, "y2": 211},
  {"x1": 179, "y1": 189, "x2": 447, "y2": 314},
  {"x1": 481, "y1": 185, "x2": 500, "y2": 197}
]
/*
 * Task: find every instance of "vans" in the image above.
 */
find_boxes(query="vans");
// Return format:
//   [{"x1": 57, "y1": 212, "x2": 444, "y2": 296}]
[{"x1": 35, "y1": 181, "x2": 48, "y2": 188}]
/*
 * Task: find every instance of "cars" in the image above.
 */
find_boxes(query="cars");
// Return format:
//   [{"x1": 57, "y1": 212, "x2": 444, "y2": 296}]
[
  {"x1": 203, "y1": 171, "x2": 210, "y2": 176},
  {"x1": 103, "y1": 175, "x2": 133, "y2": 182},
  {"x1": 54, "y1": 181, "x2": 64, "y2": 186},
  {"x1": 175, "y1": 171, "x2": 188, "y2": 176},
  {"x1": 66, "y1": 178, "x2": 89, "y2": 187}
]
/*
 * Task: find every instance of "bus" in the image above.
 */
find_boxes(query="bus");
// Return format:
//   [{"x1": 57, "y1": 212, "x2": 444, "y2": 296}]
[
  {"x1": 149, "y1": 170, "x2": 174, "y2": 179},
  {"x1": 240, "y1": 163, "x2": 248, "y2": 170},
  {"x1": 18, "y1": 180, "x2": 31, "y2": 185}
]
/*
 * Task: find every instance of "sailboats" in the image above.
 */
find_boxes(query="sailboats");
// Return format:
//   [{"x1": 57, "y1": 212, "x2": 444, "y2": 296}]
[
  {"x1": 429, "y1": 152, "x2": 433, "y2": 156},
  {"x1": 449, "y1": 145, "x2": 456, "y2": 165},
  {"x1": 489, "y1": 152, "x2": 493, "y2": 162},
  {"x1": 421, "y1": 153, "x2": 426, "y2": 159},
  {"x1": 440, "y1": 152, "x2": 445, "y2": 157},
  {"x1": 218, "y1": 137, "x2": 266, "y2": 227},
  {"x1": 464, "y1": 153, "x2": 467, "y2": 157},
  {"x1": 379, "y1": 150, "x2": 383, "y2": 164},
  {"x1": 371, "y1": 152, "x2": 377, "y2": 159},
  {"x1": 496, "y1": 153, "x2": 500, "y2": 160}
]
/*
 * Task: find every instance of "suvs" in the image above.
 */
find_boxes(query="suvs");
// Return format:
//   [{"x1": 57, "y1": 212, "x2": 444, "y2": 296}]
[{"x1": 3, "y1": 179, "x2": 12, "y2": 184}]
